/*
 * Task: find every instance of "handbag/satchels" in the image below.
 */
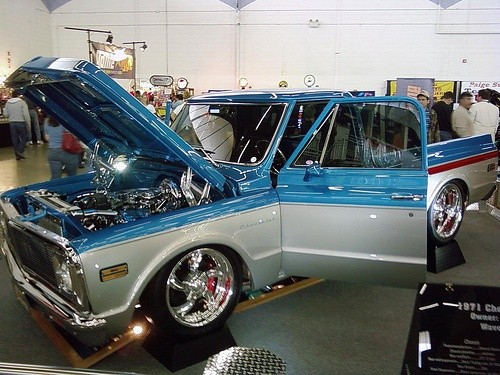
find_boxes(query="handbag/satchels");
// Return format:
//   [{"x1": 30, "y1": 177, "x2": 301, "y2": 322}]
[{"x1": 62, "y1": 131, "x2": 82, "y2": 154}]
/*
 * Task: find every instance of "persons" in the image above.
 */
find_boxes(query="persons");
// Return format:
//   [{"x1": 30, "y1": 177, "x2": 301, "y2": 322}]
[
  {"x1": 413, "y1": 88, "x2": 500, "y2": 144},
  {"x1": 44, "y1": 114, "x2": 82, "y2": 181},
  {"x1": 3, "y1": 90, "x2": 184, "y2": 169}
]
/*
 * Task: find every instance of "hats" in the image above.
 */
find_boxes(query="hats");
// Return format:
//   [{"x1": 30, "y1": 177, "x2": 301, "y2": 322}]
[{"x1": 416, "y1": 90, "x2": 430, "y2": 99}]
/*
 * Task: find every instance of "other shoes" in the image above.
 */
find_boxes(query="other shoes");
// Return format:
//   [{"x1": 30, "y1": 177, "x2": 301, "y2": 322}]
[{"x1": 14, "y1": 151, "x2": 26, "y2": 160}]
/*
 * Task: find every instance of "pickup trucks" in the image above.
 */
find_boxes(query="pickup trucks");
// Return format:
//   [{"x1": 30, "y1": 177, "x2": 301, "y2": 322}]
[{"x1": 0, "y1": 55, "x2": 499, "y2": 346}]
